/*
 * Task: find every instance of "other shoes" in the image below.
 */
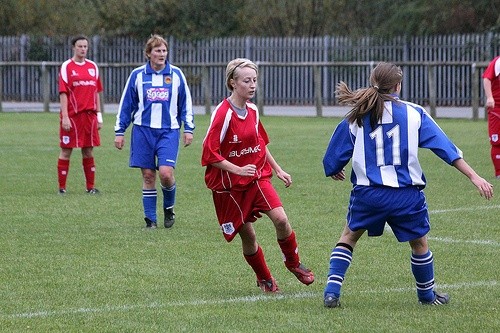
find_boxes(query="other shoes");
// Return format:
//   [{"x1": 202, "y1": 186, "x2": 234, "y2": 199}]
[
  {"x1": 86, "y1": 189, "x2": 99, "y2": 193},
  {"x1": 59, "y1": 191, "x2": 66, "y2": 194}
]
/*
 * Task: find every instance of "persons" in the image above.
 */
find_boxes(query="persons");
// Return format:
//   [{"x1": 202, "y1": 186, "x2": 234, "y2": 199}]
[
  {"x1": 113, "y1": 34, "x2": 195, "y2": 229},
  {"x1": 482, "y1": 56, "x2": 500, "y2": 178},
  {"x1": 322, "y1": 62, "x2": 494, "y2": 307},
  {"x1": 58, "y1": 34, "x2": 104, "y2": 194},
  {"x1": 201, "y1": 58, "x2": 315, "y2": 292}
]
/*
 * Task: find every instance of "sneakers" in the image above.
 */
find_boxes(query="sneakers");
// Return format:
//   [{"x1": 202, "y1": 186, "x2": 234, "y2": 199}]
[
  {"x1": 257, "y1": 277, "x2": 278, "y2": 293},
  {"x1": 419, "y1": 290, "x2": 448, "y2": 305},
  {"x1": 163, "y1": 205, "x2": 175, "y2": 227},
  {"x1": 284, "y1": 259, "x2": 314, "y2": 285},
  {"x1": 324, "y1": 293, "x2": 338, "y2": 307},
  {"x1": 144, "y1": 217, "x2": 157, "y2": 229}
]
